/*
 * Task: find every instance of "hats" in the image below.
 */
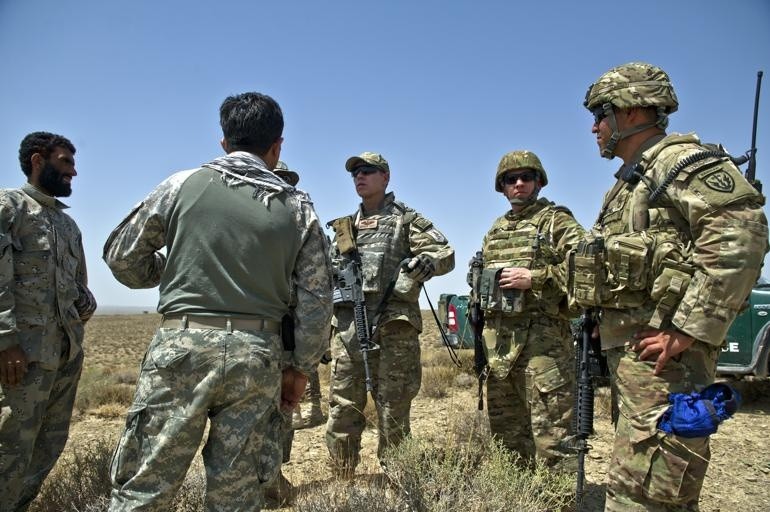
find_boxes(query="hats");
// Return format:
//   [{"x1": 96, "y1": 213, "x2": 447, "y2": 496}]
[
  {"x1": 271, "y1": 160, "x2": 300, "y2": 187},
  {"x1": 345, "y1": 152, "x2": 390, "y2": 175}
]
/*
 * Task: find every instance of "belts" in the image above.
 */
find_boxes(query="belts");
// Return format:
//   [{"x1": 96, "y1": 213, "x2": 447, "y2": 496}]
[{"x1": 159, "y1": 312, "x2": 280, "y2": 336}]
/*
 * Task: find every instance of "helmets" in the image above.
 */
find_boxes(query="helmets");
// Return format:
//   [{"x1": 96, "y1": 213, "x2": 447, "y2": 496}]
[
  {"x1": 582, "y1": 61, "x2": 680, "y2": 116},
  {"x1": 494, "y1": 149, "x2": 548, "y2": 192}
]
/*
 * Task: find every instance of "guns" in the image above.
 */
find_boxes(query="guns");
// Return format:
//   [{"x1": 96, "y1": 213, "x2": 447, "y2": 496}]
[
  {"x1": 469, "y1": 251, "x2": 483, "y2": 411},
  {"x1": 326, "y1": 217, "x2": 375, "y2": 393},
  {"x1": 573, "y1": 308, "x2": 608, "y2": 510},
  {"x1": 745, "y1": 70, "x2": 764, "y2": 193}
]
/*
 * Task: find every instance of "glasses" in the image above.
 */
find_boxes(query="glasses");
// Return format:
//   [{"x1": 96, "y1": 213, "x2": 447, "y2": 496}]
[
  {"x1": 351, "y1": 164, "x2": 377, "y2": 176},
  {"x1": 594, "y1": 111, "x2": 608, "y2": 126},
  {"x1": 502, "y1": 172, "x2": 534, "y2": 184}
]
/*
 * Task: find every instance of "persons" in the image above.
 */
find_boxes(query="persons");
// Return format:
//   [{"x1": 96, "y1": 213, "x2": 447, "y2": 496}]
[
  {"x1": 272, "y1": 161, "x2": 328, "y2": 429},
  {"x1": 581, "y1": 63, "x2": 769, "y2": 511},
  {"x1": 470, "y1": 149, "x2": 590, "y2": 487},
  {"x1": 100, "y1": 92, "x2": 334, "y2": 511},
  {"x1": 0, "y1": 131, "x2": 95, "y2": 511},
  {"x1": 327, "y1": 150, "x2": 455, "y2": 493}
]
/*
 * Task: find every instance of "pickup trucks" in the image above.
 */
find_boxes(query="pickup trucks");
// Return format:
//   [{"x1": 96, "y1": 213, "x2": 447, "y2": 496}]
[
  {"x1": 437, "y1": 292, "x2": 475, "y2": 351},
  {"x1": 711, "y1": 274, "x2": 769, "y2": 394}
]
